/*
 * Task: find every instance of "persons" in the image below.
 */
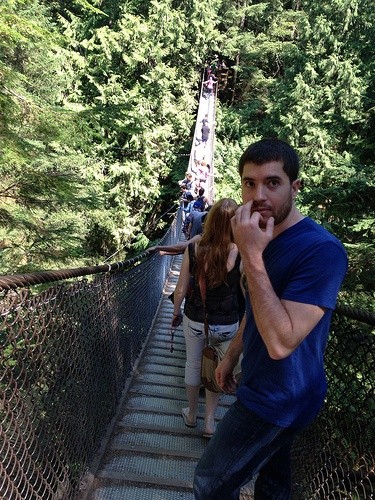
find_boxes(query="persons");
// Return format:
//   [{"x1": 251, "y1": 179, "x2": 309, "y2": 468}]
[
  {"x1": 193, "y1": 138, "x2": 349, "y2": 500},
  {"x1": 202, "y1": 69, "x2": 219, "y2": 99},
  {"x1": 172, "y1": 198, "x2": 245, "y2": 438},
  {"x1": 146, "y1": 114, "x2": 217, "y2": 255}
]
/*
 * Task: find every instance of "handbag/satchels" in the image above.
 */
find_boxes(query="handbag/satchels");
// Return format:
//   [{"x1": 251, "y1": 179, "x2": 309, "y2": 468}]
[
  {"x1": 204, "y1": 339, "x2": 244, "y2": 393},
  {"x1": 187, "y1": 214, "x2": 192, "y2": 233}
]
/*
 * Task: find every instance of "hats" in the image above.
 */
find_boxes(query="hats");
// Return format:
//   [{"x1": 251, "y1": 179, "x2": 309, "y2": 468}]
[{"x1": 193, "y1": 201, "x2": 201, "y2": 209}]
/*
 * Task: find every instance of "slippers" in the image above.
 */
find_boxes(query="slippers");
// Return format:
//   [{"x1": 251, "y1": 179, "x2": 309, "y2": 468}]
[
  {"x1": 202, "y1": 434, "x2": 213, "y2": 438},
  {"x1": 182, "y1": 407, "x2": 197, "y2": 428}
]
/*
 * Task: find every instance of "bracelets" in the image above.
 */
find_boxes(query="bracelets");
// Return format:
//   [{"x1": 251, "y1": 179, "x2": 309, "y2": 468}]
[{"x1": 173, "y1": 310, "x2": 181, "y2": 316}]
